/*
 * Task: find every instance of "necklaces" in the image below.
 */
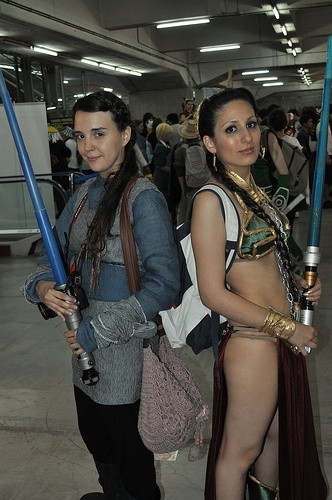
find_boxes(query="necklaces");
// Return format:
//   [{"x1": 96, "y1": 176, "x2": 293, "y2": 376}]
[{"x1": 252, "y1": 184, "x2": 301, "y2": 316}]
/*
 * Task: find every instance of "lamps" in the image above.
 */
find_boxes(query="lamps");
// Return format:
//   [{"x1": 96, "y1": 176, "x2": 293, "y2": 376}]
[{"x1": 28, "y1": 0, "x2": 314, "y2": 88}]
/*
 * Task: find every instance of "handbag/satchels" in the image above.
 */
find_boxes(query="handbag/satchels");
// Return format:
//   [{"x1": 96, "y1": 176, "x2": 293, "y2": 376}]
[{"x1": 137, "y1": 335, "x2": 212, "y2": 454}]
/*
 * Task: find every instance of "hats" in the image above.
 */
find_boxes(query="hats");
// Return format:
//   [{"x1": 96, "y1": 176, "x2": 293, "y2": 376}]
[{"x1": 177, "y1": 120, "x2": 199, "y2": 139}]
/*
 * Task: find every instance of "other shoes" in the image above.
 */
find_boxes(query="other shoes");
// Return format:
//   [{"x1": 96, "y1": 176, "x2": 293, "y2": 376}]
[{"x1": 294, "y1": 212, "x2": 299, "y2": 217}]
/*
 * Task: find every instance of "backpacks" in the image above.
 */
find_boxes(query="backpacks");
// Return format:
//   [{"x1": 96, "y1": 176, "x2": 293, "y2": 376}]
[
  {"x1": 259, "y1": 125, "x2": 309, "y2": 196},
  {"x1": 181, "y1": 141, "x2": 212, "y2": 188},
  {"x1": 157, "y1": 184, "x2": 240, "y2": 368}
]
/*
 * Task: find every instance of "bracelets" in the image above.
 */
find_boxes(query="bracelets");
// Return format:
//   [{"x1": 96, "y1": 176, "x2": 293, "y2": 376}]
[{"x1": 262, "y1": 306, "x2": 296, "y2": 340}]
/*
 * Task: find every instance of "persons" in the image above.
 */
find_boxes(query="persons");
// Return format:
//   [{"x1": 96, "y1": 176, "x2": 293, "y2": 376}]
[
  {"x1": 49, "y1": 97, "x2": 332, "y2": 229},
  {"x1": 187, "y1": 86, "x2": 323, "y2": 500},
  {"x1": 19, "y1": 92, "x2": 182, "y2": 500}
]
